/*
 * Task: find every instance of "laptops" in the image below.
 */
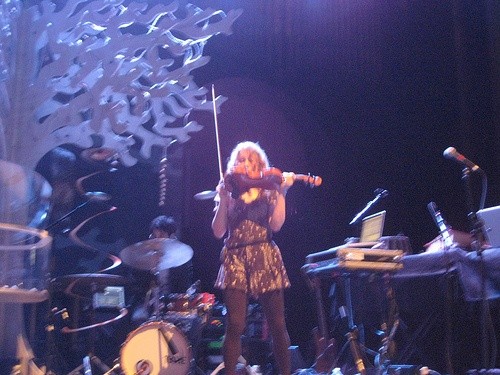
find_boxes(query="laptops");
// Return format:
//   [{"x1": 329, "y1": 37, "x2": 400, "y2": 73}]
[{"x1": 328, "y1": 210, "x2": 386, "y2": 248}]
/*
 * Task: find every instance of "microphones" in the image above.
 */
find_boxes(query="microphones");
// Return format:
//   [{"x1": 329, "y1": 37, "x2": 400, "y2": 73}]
[
  {"x1": 442, "y1": 146, "x2": 481, "y2": 172},
  {"x1": 160, "y1": 327, "x2": 184, "y2": 363}
]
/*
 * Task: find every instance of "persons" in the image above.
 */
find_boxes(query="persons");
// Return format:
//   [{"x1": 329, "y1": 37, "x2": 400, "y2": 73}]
[
  {"x1": 129, "y1": 215, "x2": 195, "y2": 326},
  {"x1": 208, "y1": 141, "x2": 296, "y2": 375}
]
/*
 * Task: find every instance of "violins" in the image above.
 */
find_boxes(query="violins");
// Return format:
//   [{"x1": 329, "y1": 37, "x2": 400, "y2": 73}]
[{"x1": 224, "y1": 166, "x2": 323, "y2": 198}]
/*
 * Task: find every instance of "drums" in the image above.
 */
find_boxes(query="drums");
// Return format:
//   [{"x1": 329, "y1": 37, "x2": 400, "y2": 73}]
[
  {"x1": 161, "y1": 293, "x2": 198, "y2": 320},
  {"x1": 119, "y1": 321, "x2": 195, "y2": 375}
]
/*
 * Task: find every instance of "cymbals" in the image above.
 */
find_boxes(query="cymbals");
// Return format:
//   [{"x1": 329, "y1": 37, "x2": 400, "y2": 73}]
[
  {"x1": 120, "y1": 238, "x2": 193, "y2": 270},
  {"x1": 54, "y1": 273, "x2": 124, "y2": 286}
]
